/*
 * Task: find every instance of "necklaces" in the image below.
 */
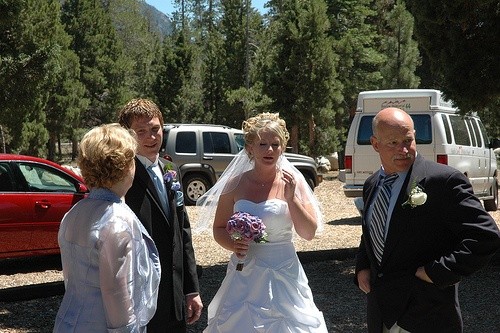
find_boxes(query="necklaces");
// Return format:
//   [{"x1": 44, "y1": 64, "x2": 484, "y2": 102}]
[{"x1": 254, "y1": 170, "x2": 277, "y2": 187}]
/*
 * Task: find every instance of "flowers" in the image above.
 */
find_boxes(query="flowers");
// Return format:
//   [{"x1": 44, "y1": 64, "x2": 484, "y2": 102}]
[
  {"x1": 160, "y1": 164, "x2": 177, "y2": 181},
  {"x1": 226, "y1": 212, "x2": 269, "y2": 247},
  {"x1": 401, "y1": 177, "x2": 427, "y2": 209}
]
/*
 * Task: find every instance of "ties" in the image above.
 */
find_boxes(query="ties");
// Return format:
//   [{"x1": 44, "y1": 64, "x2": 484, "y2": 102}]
[
  {"x1": 146, "y1": 162, "x2": 170, "y2": 218},
  {"x1": 368, "y1": 172, "x2": 400, "y2": 267}
]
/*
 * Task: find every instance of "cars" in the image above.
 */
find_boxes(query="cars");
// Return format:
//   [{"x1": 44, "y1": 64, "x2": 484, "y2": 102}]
[{"x1": 0, "y1": 152, "x2": 88, "y2": 259}]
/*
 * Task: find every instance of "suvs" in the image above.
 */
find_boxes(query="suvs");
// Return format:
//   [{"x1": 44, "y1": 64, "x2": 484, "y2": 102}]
[{"x1": 159, "y1": 123, "x2": 324, "y2": 205}]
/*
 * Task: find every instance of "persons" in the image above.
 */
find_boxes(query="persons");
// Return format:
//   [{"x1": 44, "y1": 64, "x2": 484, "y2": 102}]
[
  {"x1": 354, "y1": 106, "x2": 500, "y2": 333},
  {"x1": 53, "y1": 123, "x2": 161, "y2": 333},
  {"x1": 192, "y1": 112, "x2": 329, "y2": 333},
  {"x1": 118, "y1": 97, "x2": 202, "y2": 333}
]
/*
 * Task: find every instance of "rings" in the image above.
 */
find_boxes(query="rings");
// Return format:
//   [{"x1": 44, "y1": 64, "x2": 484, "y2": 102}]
[{"x1": 289, "y1": 177, "x2": 292, "y2": 182}]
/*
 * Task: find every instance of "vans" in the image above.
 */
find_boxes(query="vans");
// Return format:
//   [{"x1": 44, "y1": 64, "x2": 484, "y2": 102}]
[{"x1": 341, "y1": 89, "x2": 499, "y2": 215}]
